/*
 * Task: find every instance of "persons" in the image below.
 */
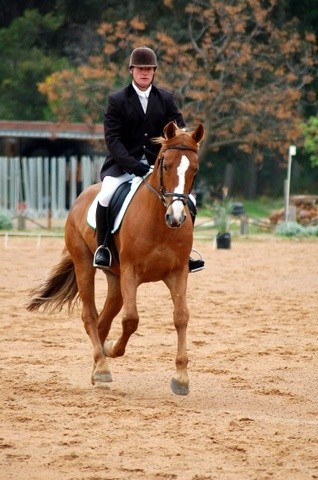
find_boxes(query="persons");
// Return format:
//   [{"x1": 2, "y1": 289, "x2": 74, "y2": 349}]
[{"x1": 93, "y1": 47, "x2": 205, "y2": 273}]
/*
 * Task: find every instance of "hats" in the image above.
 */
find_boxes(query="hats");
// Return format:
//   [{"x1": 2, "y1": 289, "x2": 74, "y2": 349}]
[{"x1": 129, "y1": 47, "x2": 157, "y2": 70}]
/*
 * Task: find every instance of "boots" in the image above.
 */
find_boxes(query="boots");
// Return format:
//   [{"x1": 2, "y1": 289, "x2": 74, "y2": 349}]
[
  {"x1": 96, "y1": 201, "x2": 110, "y2": 265},
  {"x1": 189, "y1": 259, "x2": 204, "y2": 270}
]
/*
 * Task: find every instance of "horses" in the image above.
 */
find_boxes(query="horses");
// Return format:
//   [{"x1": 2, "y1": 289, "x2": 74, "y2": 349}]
[{"x1": 26, "y1": 121, "x2": 206, "y2": 396}]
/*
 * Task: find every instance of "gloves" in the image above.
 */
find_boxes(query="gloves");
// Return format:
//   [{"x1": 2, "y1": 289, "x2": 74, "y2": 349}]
[{"x1": 133, "y1": 162, "x2": 150, "y2": 177}]
